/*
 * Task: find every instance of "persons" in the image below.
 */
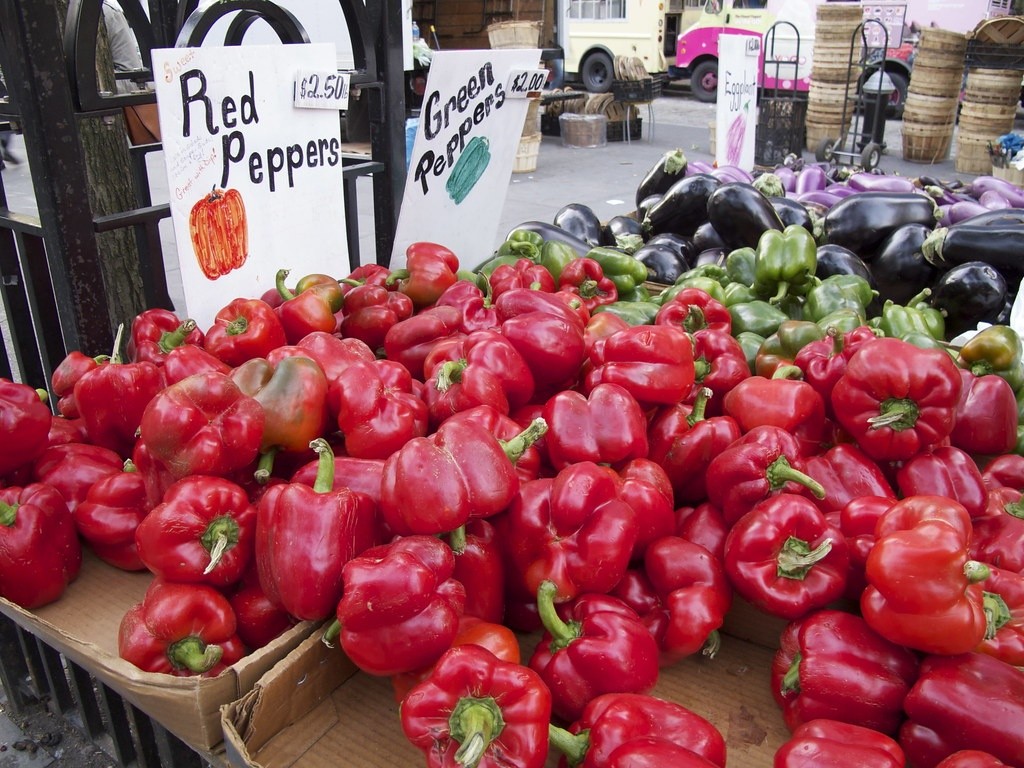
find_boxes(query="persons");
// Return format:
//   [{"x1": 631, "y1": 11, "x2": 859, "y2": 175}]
[{"x1": 102, "y1": 0, "x2": 149, "y2": 94}]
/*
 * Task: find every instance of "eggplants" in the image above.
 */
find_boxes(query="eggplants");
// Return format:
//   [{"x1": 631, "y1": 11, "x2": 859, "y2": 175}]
[{"x1": 471, "y1": 153, "x2": 1024, "y2": 345}]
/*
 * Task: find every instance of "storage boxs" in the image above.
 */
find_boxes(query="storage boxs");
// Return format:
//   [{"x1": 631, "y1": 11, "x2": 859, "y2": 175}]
[{"x1": 1, "y1": 1, "x2": 1024, "y2": 767}]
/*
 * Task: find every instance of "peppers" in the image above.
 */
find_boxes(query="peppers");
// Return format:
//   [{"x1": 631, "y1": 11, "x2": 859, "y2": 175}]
[{"x1": 0, "y1": 223, "x2": 1024, "y2": 768}]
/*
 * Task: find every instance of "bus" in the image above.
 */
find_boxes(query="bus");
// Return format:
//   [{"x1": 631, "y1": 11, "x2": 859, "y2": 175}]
[
  {"x1": 669, "y1": 1, "x2": 1014, "y2": 105},
  {"x1": 552, "y1": 1, "x2": 707, "y2": 95}
]
